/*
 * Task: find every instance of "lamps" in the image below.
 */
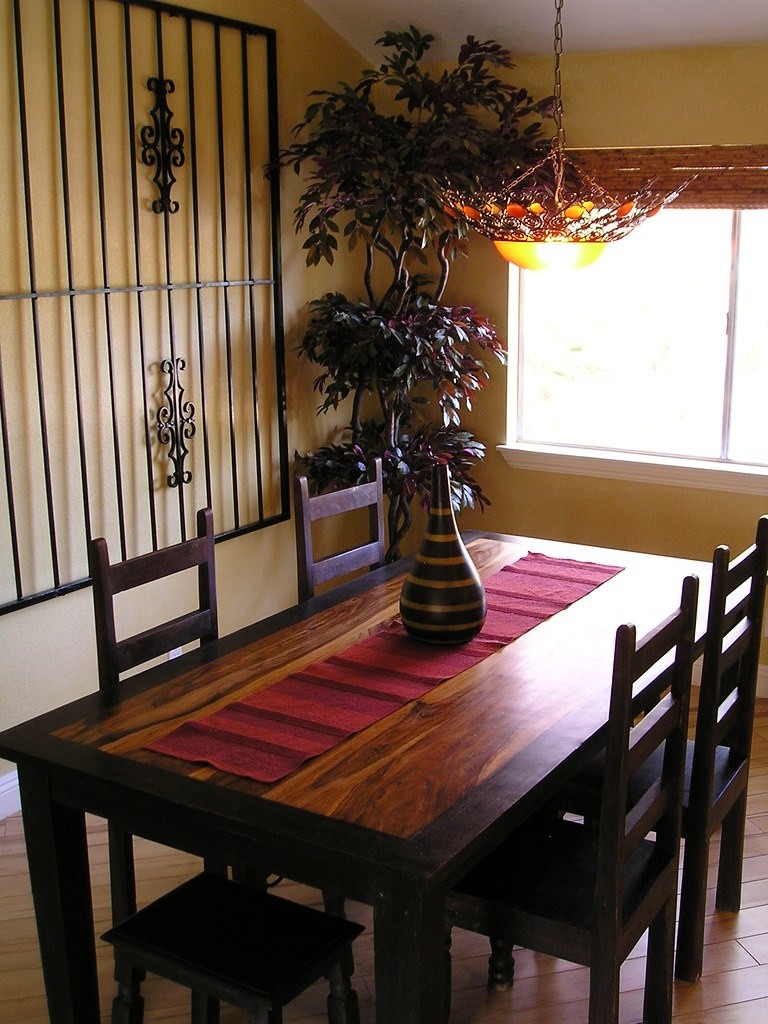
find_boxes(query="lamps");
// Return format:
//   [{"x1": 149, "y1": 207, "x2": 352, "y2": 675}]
[{"x1": 423, "y1": 1, "x2": 698, "y2": 272}]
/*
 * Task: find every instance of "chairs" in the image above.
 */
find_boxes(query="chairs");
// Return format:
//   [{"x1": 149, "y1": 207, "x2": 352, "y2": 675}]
[{"x1": 85, "y1": 457, "x2": 768, "y2": 1023}]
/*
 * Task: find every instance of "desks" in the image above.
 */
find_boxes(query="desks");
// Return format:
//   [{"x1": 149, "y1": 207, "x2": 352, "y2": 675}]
[{"x1": 1, "y1": 529, "x2": 753, "y2": 1021}]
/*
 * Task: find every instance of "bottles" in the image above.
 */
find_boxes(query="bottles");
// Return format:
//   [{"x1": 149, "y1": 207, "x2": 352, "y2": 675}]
[{"x1": 399, "y1": 463, "x2": 487, "y2": 648}]
